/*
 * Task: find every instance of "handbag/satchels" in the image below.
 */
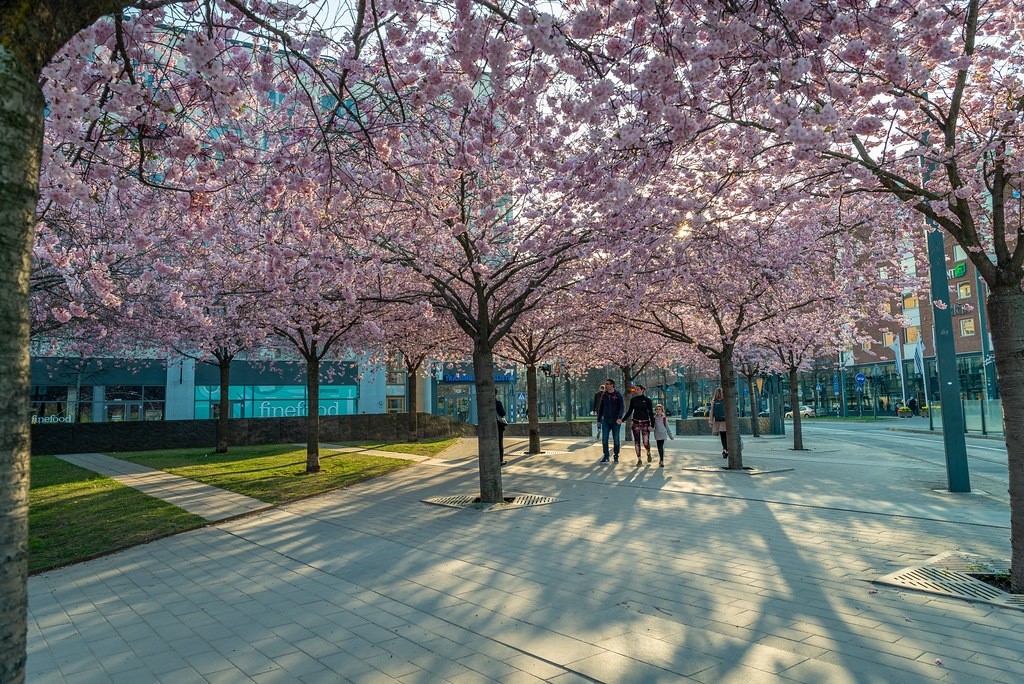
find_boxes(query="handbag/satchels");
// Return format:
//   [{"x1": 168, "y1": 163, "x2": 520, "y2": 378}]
[{"x1": 497, "y1": 418, "x2": 509, "y2": 427}]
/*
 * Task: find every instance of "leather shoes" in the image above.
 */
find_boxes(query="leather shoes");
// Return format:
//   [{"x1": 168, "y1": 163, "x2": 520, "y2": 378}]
[{"x1": 501, "y1": 461, "x2": 508, "y2": 466}]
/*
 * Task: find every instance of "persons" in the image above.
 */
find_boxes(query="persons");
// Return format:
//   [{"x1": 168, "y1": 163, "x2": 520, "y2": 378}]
[
  {"x1": 593, "y1": 378, "x2": 674, "y2": 468},
  {"x1": 494, "y1": 388, "x2": 508, "y2": 465},
  {"x1": 708, "y1": 387, "x2": 729, "y2": 459}
]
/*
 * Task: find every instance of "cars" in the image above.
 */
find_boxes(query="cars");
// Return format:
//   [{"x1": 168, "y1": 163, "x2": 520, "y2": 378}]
[
  {"x1": 758, "y1": 408, "x2": 770, "y2": 418},
  {"x1": 785, "y1": 405, "x2": 817, "y2": 418},
  {"x1": 693, "y1": 406, "x2": 711, "y2": 417}
]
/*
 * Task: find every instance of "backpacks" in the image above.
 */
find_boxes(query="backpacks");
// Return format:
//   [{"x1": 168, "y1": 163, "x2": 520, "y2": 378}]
[{"x1": 713, "y1": 399, "x2": 725, "y2": 422}]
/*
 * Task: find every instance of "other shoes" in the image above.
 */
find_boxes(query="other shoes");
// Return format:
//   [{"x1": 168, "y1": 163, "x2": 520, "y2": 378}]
[
  {"x1": 722, "y1": 451, "x2": 728, "y2": 460},
  {"x1": 597, "y1": 429, "x2": 601, "y2": 439}
]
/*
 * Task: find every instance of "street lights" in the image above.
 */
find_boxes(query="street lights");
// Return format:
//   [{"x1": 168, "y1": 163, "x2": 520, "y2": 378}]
[
  {"x1": 539, "y1": 355, "x2": 566, "y2": 422},
  {"x1": 655, "y1": 365, "x2": 689, "y2": 419}
]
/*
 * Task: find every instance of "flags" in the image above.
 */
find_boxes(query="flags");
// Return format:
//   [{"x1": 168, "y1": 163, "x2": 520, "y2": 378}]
[
  {"x1": 914, "y1": 336, "x2": 926, "y2": 374},
  {"x1": 888, "y1": 337, "x2": 901, "y2": 375}
]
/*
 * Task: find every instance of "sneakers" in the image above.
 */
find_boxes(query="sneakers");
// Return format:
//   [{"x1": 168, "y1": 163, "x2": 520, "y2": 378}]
[
  {"x1": 659, "y1": 461, "x2": 665, "y2": 468},
  {"x1": 636, "y1": 460, "x2": 643, "y2": 468},
  {"x1": 645, "y1": 454, "x2": 653, "y2": 462},
  {"x1": 600, "y1": 456, "x2": 612, "y2": 464},
  {"x1": 613, "y1": 456, "x2": 621, "y2": 464}
]
